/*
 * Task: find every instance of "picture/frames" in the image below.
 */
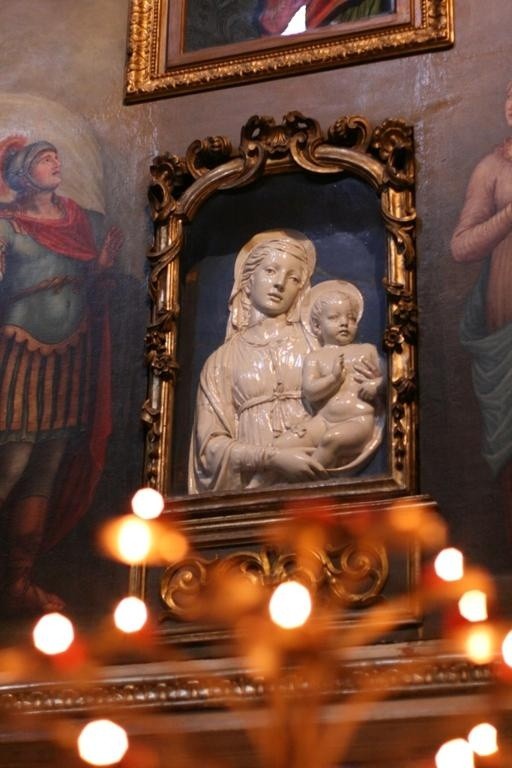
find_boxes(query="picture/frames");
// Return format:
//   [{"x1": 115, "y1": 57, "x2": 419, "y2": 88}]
[
  {"x1": 123, "y1": 1, "x2": 454, "y2": 107},
  {"x1": 127, "y1": 110, "x2": 422, "y2": 646}
]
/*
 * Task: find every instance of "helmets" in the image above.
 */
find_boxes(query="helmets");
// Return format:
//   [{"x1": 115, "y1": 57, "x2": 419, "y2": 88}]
[{"x1": 7, "y1": 140, "x2": 58, "y2": 192}]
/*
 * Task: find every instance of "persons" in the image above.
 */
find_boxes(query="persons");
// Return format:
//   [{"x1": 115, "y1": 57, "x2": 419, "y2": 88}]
[
  {"x1": 0, "y1": 135, "x2": 123, "y2": 615},
  {"x1": 244, "y1": 279, "x2": 389, "y2": 490},
  {"x1": 451, "y1": 79, "x2": 512, "y2": 547},
  {"x1": 187, "y1": 228, "x2": 388, "y2": 495}
]
